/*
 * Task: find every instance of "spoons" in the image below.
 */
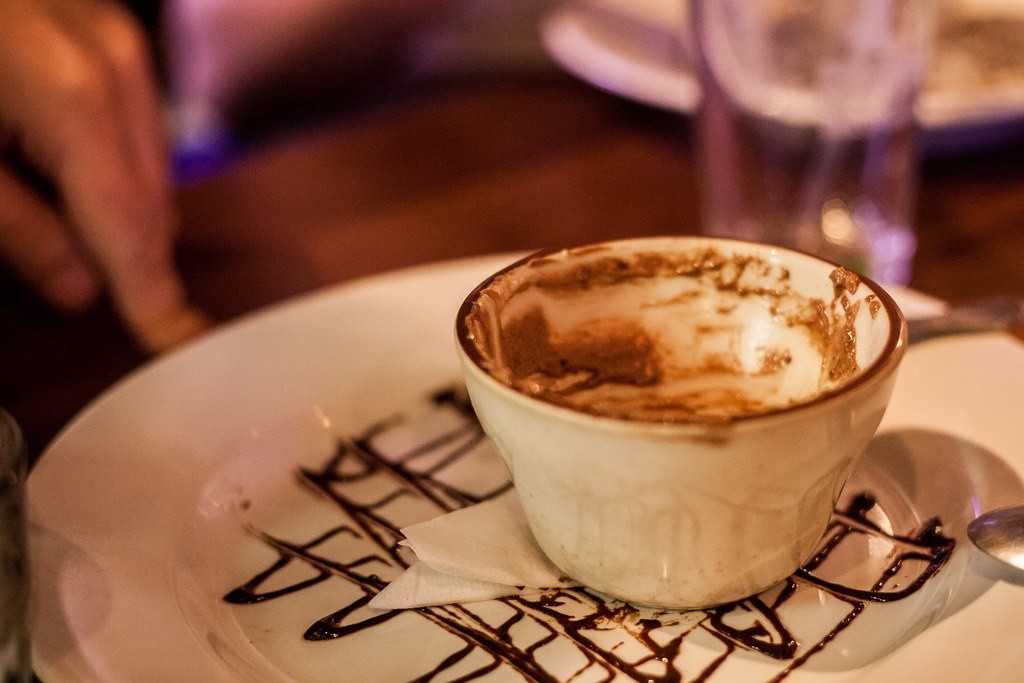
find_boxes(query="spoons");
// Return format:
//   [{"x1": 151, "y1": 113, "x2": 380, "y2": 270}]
[{"x1": 966, "y1": 503, "x2": 1024, "y2": 571}]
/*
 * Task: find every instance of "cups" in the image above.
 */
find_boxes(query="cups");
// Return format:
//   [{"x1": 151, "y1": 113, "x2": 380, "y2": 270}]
[
  {"x1": 693, "y1": 1, "x2": 933, "y2": 425},
  {"x1": 452, "y1": 232, "x2": 910, "y2": 617}
]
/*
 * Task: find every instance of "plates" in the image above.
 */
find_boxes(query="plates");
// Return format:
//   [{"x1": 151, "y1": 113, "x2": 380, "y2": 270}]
[
  {"x1": 537, "y1": 0, "x2": 1024, "y2": 131},
  {"x1": 18, "y1": 249, "x2": 1024, "y2": 683}
]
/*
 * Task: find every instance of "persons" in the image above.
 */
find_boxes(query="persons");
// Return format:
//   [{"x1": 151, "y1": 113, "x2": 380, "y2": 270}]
[{"x1": 0, "y1": 0, "x2": 215, "y2": 349}]
